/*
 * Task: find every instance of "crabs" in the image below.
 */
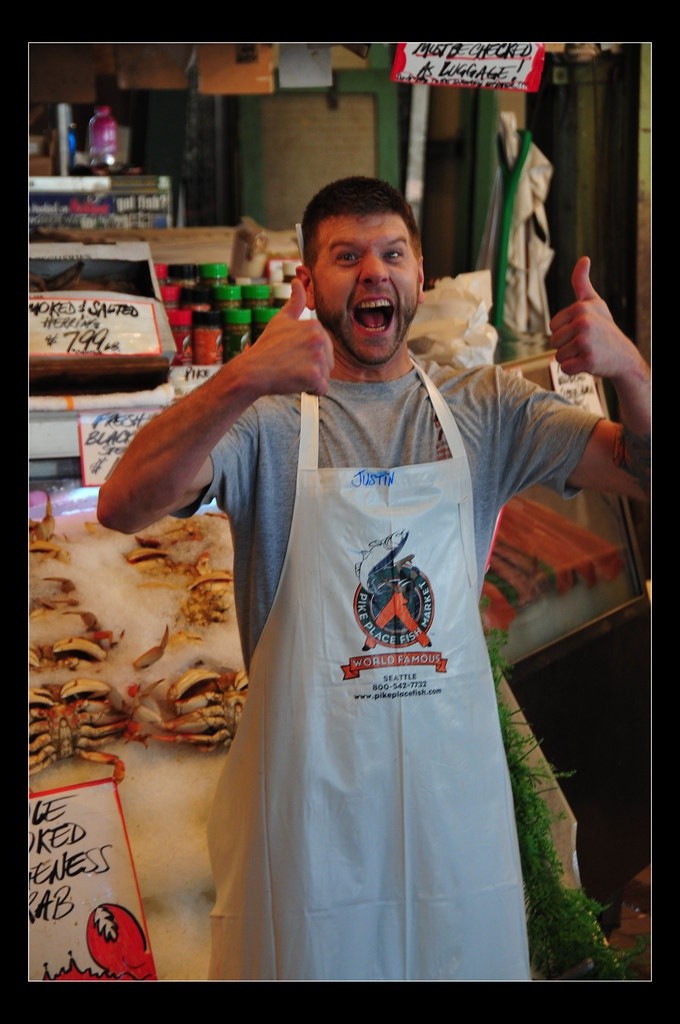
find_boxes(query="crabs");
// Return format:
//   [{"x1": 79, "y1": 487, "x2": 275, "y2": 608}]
[{"x1": 28, "y1": 496, "x2": 250, "y2": 787}]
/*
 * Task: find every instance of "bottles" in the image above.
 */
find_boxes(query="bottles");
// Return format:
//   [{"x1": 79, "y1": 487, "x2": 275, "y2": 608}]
[{"x1": 88, "y1": 106, "x2": 118, "y2": 175}]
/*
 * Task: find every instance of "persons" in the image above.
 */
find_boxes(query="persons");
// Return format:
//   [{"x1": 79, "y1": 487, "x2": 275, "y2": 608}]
[{"x1": 97, "y1": 176, "x2": 651, "y2": 981}]
